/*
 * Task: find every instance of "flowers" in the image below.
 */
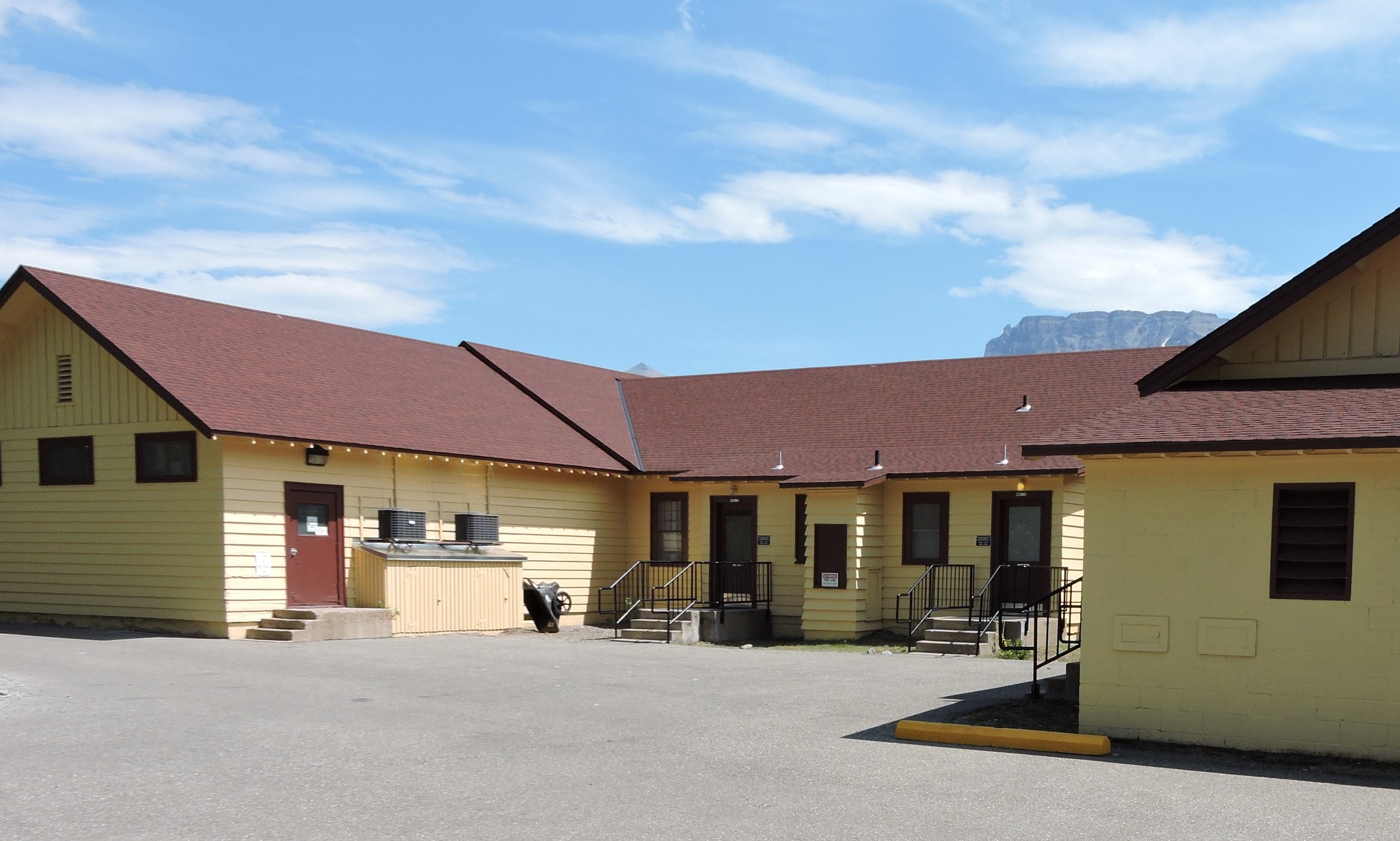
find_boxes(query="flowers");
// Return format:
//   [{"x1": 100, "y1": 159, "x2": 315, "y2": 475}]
[{"x1": 624, "y1": 595, "x2": 638, "y2": 611}]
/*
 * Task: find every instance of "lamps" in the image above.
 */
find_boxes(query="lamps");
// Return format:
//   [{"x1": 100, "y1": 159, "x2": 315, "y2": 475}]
[
  {"x1": 727, "y1": 483, "x2": 738, "y2": 495},
  {"x1": 1017, "y1": 478, "x2": 1026, "y2": 492},
  {"x1": 305, "y1": 444, "x2": 330, "y2": 467}
]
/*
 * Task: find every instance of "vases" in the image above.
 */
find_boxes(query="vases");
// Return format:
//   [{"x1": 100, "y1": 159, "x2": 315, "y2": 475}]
[{"x1": 627, "y1": 610, "x2": 638, "y2": 622}]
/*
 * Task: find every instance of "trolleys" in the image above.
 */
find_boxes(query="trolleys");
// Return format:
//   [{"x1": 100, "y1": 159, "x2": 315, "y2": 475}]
[{"x1": 523, "y1": 577, "x2": 572, "y2": 634}]
